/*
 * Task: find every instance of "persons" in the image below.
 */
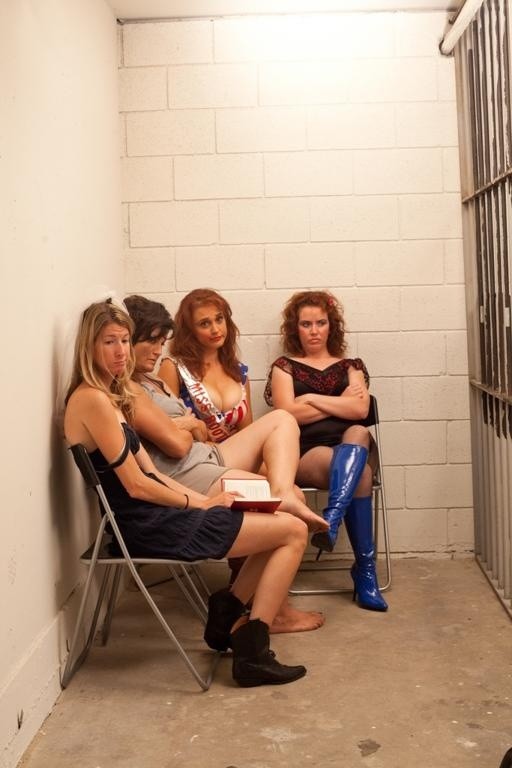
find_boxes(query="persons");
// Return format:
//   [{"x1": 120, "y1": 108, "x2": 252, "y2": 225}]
[
  {"x1": 63, "y1": 301, "x2": 306, "y2": 688},
  {"x1": 263, "y1": 290, "x2": 388, "y2": 612},
  {"x1": 157, "y1": 289, "x2": 253, "y2": 591},
  {"x1": 122, "y1": 296, "x2": 331, "y2": 633}
]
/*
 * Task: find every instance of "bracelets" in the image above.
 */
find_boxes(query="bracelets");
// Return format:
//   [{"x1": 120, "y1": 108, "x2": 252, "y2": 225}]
[{"x1": 184, "y1": 493, "x2": 189, "y2": 511}]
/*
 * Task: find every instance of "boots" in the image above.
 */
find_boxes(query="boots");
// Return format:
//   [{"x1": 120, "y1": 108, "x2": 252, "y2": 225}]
[
  {"x1": 343, "y1": 496, "x2": 388, "y2": 611},
  {"x1": 311, "y1": 443, "x2": 369, "y2": 561},
  {"x1": 204, "y1": 591, "x2": 246, "y2": 652},
  {"x1": 229, "y1": 619, "x2": 307, "y2": 688}
]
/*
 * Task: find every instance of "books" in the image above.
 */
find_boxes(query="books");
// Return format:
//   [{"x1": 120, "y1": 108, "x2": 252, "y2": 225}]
[{"x1": 221, "y1": 477, "x2": 283, "y2": 514}]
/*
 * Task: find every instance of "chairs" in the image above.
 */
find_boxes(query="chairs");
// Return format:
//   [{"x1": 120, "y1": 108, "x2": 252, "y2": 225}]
[
  {"x1": 59, "y1": 443, "x2": 226, "y2": 689},
  {"x1": 274, "y1": 396, "x2": 392, "y2": 596}
]
